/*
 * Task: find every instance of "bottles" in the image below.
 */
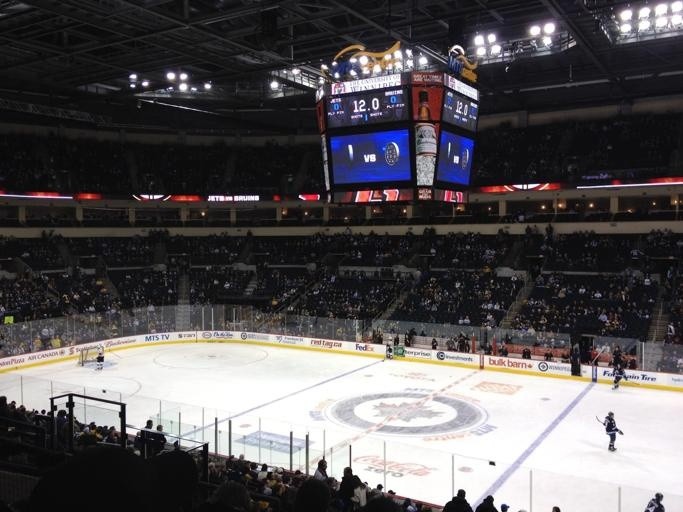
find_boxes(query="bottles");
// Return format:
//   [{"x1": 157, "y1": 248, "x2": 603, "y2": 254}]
[{"x1": 414, "y1": 92, "x2": 438, "y2": 185}]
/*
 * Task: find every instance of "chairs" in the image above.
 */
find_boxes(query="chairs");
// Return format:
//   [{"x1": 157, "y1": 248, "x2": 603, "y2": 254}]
[
  {"x1": 0, "y1": 195, "x2": 683, "y2": 376},
  {"x1": 0, "y1": 409, "x2": 466, "y2": 512}
]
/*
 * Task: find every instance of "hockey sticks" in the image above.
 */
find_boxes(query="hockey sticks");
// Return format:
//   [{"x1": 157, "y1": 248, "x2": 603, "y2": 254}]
[{"x1": 596, "y1": 416, "x2": 623, "y2": 434}]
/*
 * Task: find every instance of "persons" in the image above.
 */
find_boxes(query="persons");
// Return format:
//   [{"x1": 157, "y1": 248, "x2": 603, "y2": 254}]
[
  {"x1": 1, "y1": 398, "x2": 509, "y2": 512},
  {"x1": 609, "y1": 359, "x2": 625, "y2": 389},
  {"x1": 602, "y1": 411, "x2": 624, "y2": 451},
  {"x1": 1, "y1": 123, "x2": 683, "y2": 371},
  {"x1": 642, "y1": 492, "x2": 664, "y2": 511},
  {"x1": 552, "y1": 505, "x2": 560, "y2": 512}
]
